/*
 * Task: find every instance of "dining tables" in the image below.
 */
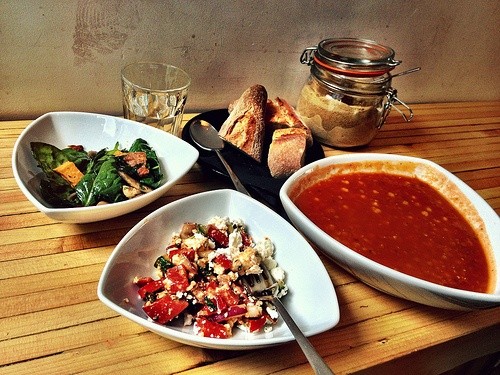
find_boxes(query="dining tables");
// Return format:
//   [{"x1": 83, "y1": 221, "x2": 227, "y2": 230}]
[{"x1": 0, "y1": 100, "x2": 500, "y2": 375}]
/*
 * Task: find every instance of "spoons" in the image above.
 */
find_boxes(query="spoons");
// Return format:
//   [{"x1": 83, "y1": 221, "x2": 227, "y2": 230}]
[{"x1": 189, "y1": 120, "x2": 255, "y2": 198}]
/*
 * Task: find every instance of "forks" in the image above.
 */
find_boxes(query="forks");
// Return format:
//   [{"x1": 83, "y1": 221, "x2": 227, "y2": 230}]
[{"x1": 242, "y1": 262, "x2": 335, "y2": 375}]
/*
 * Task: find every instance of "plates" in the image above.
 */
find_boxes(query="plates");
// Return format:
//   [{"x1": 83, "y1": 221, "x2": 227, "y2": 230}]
[
  {"x1": 182, "y1": 108, "x2": 326, "y2": 195},
  {"x1": 281, "y1": 153, "x2": 500, "y2": 310},
  {"x1": 12, "y1": 110, "x2": 200, "y2": 224},
  {"x1": 97, "y1": 189, "x2": 340, "y2": 350}
]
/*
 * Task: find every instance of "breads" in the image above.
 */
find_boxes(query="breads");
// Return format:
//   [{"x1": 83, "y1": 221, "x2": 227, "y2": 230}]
[{"x1": 218, "y1": 84, "x2": 314, "y2": 178}]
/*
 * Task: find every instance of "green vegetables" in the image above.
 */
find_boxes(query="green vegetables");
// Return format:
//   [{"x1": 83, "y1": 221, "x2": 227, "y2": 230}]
[{"x1": 29, "y1": 138, "x2": 163, "y2": 208}]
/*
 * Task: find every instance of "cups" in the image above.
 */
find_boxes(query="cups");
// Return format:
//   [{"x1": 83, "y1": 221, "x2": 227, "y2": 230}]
[{"x1": 121, "y1": 62, "x2": 192, "y2": 138}]
